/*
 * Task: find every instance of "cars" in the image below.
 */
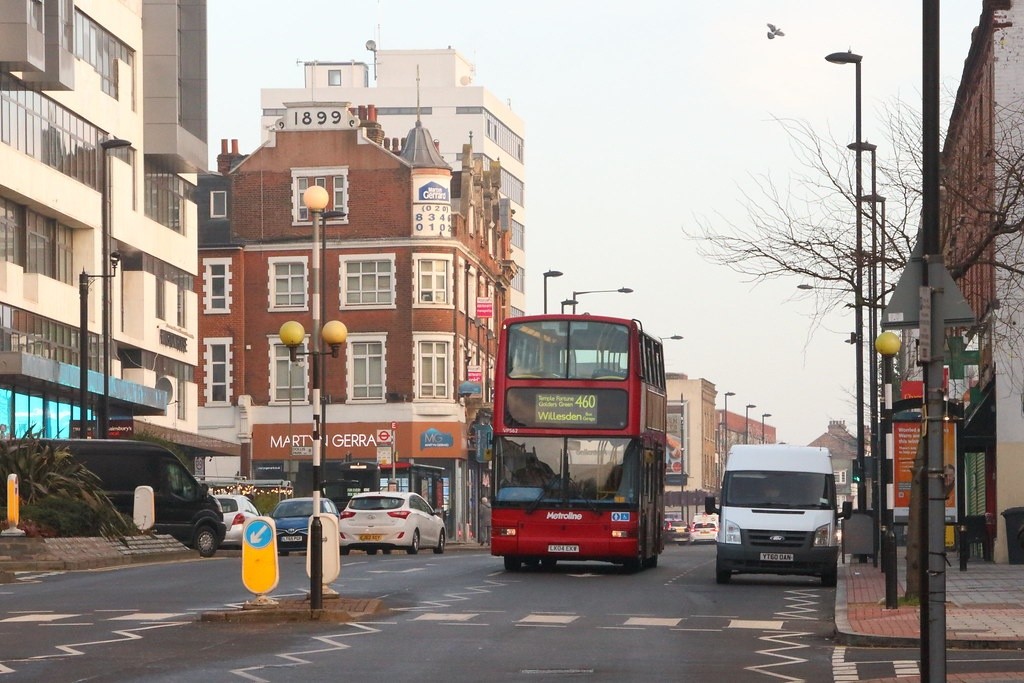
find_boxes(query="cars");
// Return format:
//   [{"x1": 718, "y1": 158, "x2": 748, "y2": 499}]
[
  {"x1": 212, "y1": 494, "x2": 264, "y2": 546},
  {"x1": 663, "y1": 511, "x2": 719, "y2": 546},
  {"x1": 268, "y1": 496, "x2": 340, "y2": 556},
  {"x1": 337, "y1": 491, "x2": 447, "y2": 556}
]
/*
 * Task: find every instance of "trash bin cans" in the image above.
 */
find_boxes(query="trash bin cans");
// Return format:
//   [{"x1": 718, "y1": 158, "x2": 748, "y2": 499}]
[
  {"x1": 893, "y1": 522, "x2": 908, "y2": 546},
  {"x1": 1001, "y1": 507, "x2": 1024, "y2": 565}
]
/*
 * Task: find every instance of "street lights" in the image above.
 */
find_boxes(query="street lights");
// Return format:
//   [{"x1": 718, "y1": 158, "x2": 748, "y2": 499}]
[
  {"x1": 316, "y1": 210, "x2": 346, "y2": 497},
  {"x1": 543, "y1": 270, "x2": 564, "y2": 315},
  {"x1": 280, "y1": 186, "x2": 349, "y2": 619},
  {"x1": 573, "y1": 287, "x2": 633, "y2": 314},
  {"x1": 79, "y1": 251, "x2": 120, "y2": 440},
  {"x1": 824, "y1": 51, "x2": 868, "y2": 564},
  {"x1": 873, "y1": 331, "x2": 902, "y2": 611},
  {"x1": 724, "y1": 392, "x2": 735, "y2": 466},
  {"x1": 561, "y1": 299, "x2": 579, "y2": 314},
  {"x1": 746, "y1": 404, "x2": 757, "y2": 444},
  {"x1": 861, "y1": 194, "x2": 886, "y2": 395},
  {"x1": 762, "y1": 413, "x2": 772, "y2": 444},
  {"x1": 847, "y1": 141, "x2": 879, "y2": 566},
  {"x1": 99, "y1": 139, "x2": 133, "y2": 440}
]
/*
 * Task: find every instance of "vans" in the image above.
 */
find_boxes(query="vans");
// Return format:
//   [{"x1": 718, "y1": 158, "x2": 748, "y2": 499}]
[
  {"x1": 705, "y1": 441, "x2": 853, "y2": 588},
  {"x1": 1, "y1": 437, "x2": 228, "y2": 559}
]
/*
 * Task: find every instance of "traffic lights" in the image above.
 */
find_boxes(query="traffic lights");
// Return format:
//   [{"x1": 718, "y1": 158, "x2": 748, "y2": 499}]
[{"x1": 852, "y1": 458, "x2": 861, "y2": 483}]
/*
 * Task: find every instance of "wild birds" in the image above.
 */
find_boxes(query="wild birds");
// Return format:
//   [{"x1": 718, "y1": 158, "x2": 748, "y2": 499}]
[{"x1": 766, "y1": 23, "x2": 785, "y2": 38}]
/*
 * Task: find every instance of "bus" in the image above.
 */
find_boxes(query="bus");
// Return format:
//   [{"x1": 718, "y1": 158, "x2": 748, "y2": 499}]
[{"x1": 485, "y1": 315, "x2": 669, "y2": 575}]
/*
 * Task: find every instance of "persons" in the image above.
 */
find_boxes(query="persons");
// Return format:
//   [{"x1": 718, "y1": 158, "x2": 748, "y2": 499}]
[
  {"x1": 479, "y1": 497, "x2": 491, "y2": 546},
  {"x1": 514, "y1": 455, "x2": 546, "y2": 478},
  {"x1": 944, "y1": 463, "x2": 955, "y2": 507}
]
